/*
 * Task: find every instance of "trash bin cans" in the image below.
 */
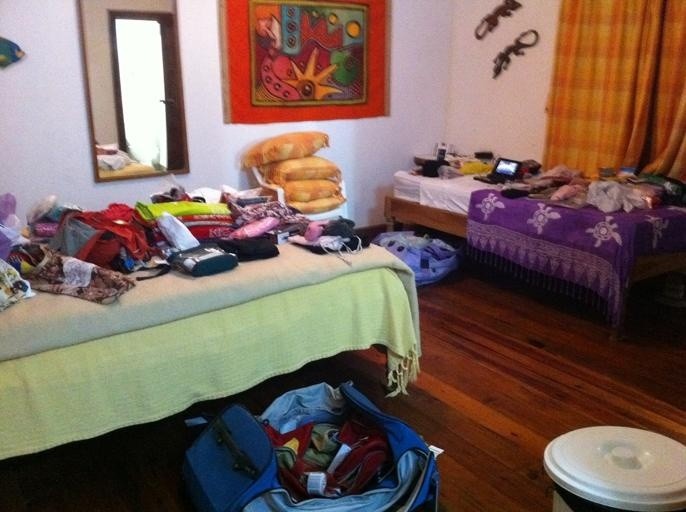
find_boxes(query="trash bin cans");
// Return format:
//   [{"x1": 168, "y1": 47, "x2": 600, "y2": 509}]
[{"x1": 543, "y1": 425, "x2": 686, "y2": 512}]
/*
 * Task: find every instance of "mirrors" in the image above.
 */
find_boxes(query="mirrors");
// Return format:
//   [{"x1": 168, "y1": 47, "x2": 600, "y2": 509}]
[{"x1": 77, "y1": 0, "x2": 191, "y2": 184}]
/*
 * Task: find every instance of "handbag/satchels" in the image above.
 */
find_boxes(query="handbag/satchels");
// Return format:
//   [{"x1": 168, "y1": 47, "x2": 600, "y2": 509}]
[{"x1": 181, "y1": 376, "x2": 442, "y2": 512}]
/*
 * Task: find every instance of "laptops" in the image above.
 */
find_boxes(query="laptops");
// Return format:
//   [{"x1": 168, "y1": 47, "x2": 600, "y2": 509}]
[{"x1": 473, "y1": 157, "x2": 523, "y2": 184}]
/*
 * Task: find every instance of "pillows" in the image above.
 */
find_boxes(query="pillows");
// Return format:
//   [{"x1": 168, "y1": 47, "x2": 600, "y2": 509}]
[
  {"x1": 239, "y1": 131, "x2": 329, "y2": 169},
  {"x1": 277, "y1": 195, "x2": 345, "y2": 214},
  {"x1": 263, "y1": 181, "x2": 338, "y2": 202},
  {"x1": 260, "y1": 157, "x2": 341, "y2": 184}
]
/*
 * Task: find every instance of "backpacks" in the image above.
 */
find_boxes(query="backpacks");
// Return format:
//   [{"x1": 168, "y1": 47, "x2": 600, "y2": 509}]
[{"x1": 373, "y1": 225, "x2": 459, "y2": 289}]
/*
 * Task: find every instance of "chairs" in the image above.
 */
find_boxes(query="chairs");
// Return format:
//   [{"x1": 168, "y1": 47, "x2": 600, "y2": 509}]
[{"x1": 252, "y1": 160, "x2": 347, "y2": 221}]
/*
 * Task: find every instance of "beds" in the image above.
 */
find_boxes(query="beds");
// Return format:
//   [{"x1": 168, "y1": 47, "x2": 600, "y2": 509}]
[
  {"x1": 0, "y1": 229, "x2": 421, "y2": 467},
  {"x1": 383, "y1": 141, "x2": 682, "y2": 335}
]
